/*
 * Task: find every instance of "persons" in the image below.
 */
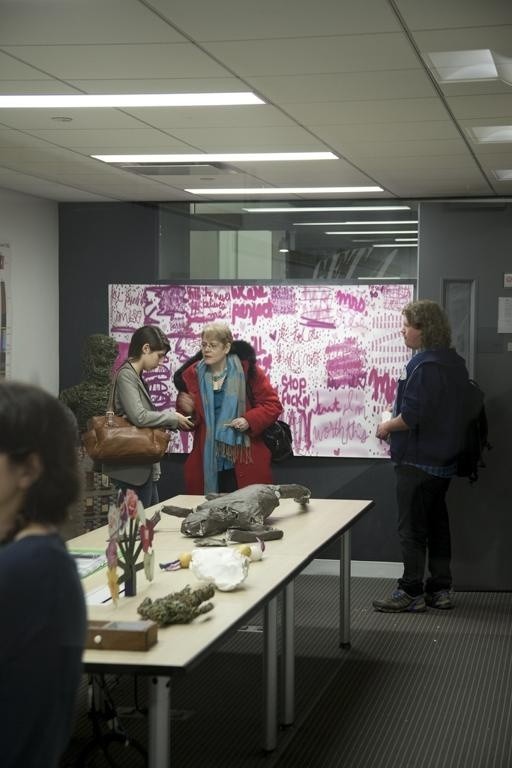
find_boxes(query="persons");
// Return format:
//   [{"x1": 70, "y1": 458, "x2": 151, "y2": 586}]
[
  {"x1": 175, "y1": 323, "x2": 282, "y2": 495},
  {"x1": 101, "y1": 327, "x2": 194, "y2": 510},
  {"x1": 1, "y1": 380, "x2": 89, "y2": 768},
  {"x1": 369, "y1": 301, "x2": 469, "y2": 614}
]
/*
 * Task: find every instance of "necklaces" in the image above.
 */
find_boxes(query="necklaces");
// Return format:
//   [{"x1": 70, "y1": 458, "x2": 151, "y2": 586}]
[{"x1": 209, "y1": 365, "x2": 227, "y2": 382}]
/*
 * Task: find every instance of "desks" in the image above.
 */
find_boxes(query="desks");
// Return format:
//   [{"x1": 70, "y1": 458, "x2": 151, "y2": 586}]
[{"x1": 66, "y1": 493, "x2": 375, "y2": 768}]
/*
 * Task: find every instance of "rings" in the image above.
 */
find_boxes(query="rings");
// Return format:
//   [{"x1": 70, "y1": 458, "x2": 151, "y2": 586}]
[{"x1": 237, "y1": 425, "x2": 239, "y2": 428}]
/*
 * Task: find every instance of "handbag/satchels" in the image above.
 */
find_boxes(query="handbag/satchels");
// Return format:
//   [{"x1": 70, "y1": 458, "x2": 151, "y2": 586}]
[
  {"x1": 81, "y1": 415, "x2": 170, "y2": 464},
  {"x1": 426, "y1": 363, "x2": 492, "y2": 479},
  {"x1": 262, "y1": 421, "x2": 293, "y2": 464}
]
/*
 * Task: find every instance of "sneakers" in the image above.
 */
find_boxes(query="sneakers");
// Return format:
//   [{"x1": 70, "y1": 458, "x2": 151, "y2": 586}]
[
  {"x1": 426, "y1": 591, "x2": 452, "y2": 609},
  {"x1": 372, "y1": 590, "x2": 425, "y2": 613}
]
